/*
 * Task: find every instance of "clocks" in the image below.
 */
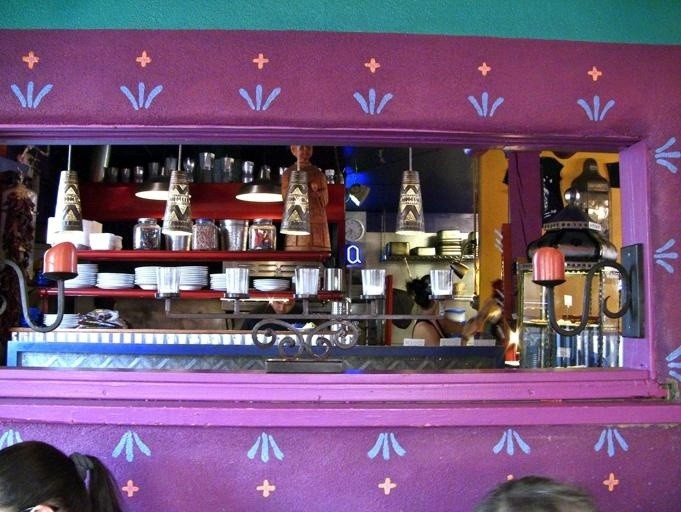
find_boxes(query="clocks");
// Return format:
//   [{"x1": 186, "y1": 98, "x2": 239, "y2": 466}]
[{"x1": 344, "y1": 218, "x2": 364, "y2": 243}]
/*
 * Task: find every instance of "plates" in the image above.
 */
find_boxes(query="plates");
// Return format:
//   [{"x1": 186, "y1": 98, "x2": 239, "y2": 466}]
[
  {"x1": 43, "y1": 311, "x2": 80, "y2": 329},
  {"x1": 62, "y1": 263, "x2": 290, "y2": 293},
  {"x1": 45, "y1": 218, "x2": 123, "y2": 251}
]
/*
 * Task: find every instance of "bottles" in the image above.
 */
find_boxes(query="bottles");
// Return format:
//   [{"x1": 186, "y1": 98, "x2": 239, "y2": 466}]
[
  {"x1": 250, "y1": 218, "x2": 278, "y2": 251},
  {"x1": 192, "y1": 218, "x2": 219, "y2": 251},
  {"x1": 218, "y1": 218, "x2": 249, "y2": 252},
  {"x1": 132, "y1": 217, "x2": 161, "y2": 250}
]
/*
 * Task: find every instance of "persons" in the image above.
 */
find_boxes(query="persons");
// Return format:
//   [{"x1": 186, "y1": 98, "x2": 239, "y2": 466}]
[
  {"x1": 281, "y1": 145, "x2": 332, "y2": 252},
  {"x1": 0, "y1": 440, "x2": 122, "y2": 512},
  {"x1": 476, "y1": 476, "x2": 597, "y2": 511},
  {"x1": 406, "y1": 275, "x2": 496, "y2": 346}
]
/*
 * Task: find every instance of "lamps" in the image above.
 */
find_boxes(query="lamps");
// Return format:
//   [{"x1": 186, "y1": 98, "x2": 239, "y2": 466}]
[
  {"x1": 449, "y1": 235, "x2": 476, "y2": 280},
  {"x1": 234, "y1": 179, "x2": 283, "y2": 204},
  {"x1": 527, "y1": 240, "x2": 648, "y2": 343},
  {"x1": 161, "y1": 144, "x2": 194, "y2": 240},
  {"x1": 135, "y1": 180, "x2": 172, "y2": 204},
  {"x1": 0, "y1": 240, "x2": 77, "y2": 336},
  {"x1": 149, "y1": 262, "x2": 461, "y2": 373},
  {"x1": 345, "y1": 183, "x2": 371, "y2": 207},
  {"x1": 51, "y1": 145, "x2": 84, "y2": 236},
  {"x1": 398, "y1": 145, "x2": 426, "y2": 239},
  {"x1": 279, "y1": 148, "x2": 313, "y2": 238}
]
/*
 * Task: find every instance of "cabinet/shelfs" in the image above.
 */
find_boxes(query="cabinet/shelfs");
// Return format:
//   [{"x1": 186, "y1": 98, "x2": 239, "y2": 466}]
[{"x1": 35, "y1": 178, "x2": 350, "y2": 303}]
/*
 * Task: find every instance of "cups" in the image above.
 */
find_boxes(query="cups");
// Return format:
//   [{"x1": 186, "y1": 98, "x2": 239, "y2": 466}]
[
  {"x1": 359, "y1": 268, "x2": 386, "y2": 296},
  {"x1": 294, "y1": 267, "x2": 319, "y2": 295},
  {"x1": 35, "y1": 271, "x2": 53, "y2": 287},
  {"x1": 21, "y1": 306, "x2": 37, "y2": 328},
  {"x1": 522, "y1": 332, "x2": 576, "y2": 370},
  {"x1": 323, "y1": 266, "x2": 345, "y2": 292},
  {"x1": 106, "y1": 149, "x2": 337, "y2": 181},
  {"x1": 429, "y1": 270, "x2": 455, "y2": 296}
]
